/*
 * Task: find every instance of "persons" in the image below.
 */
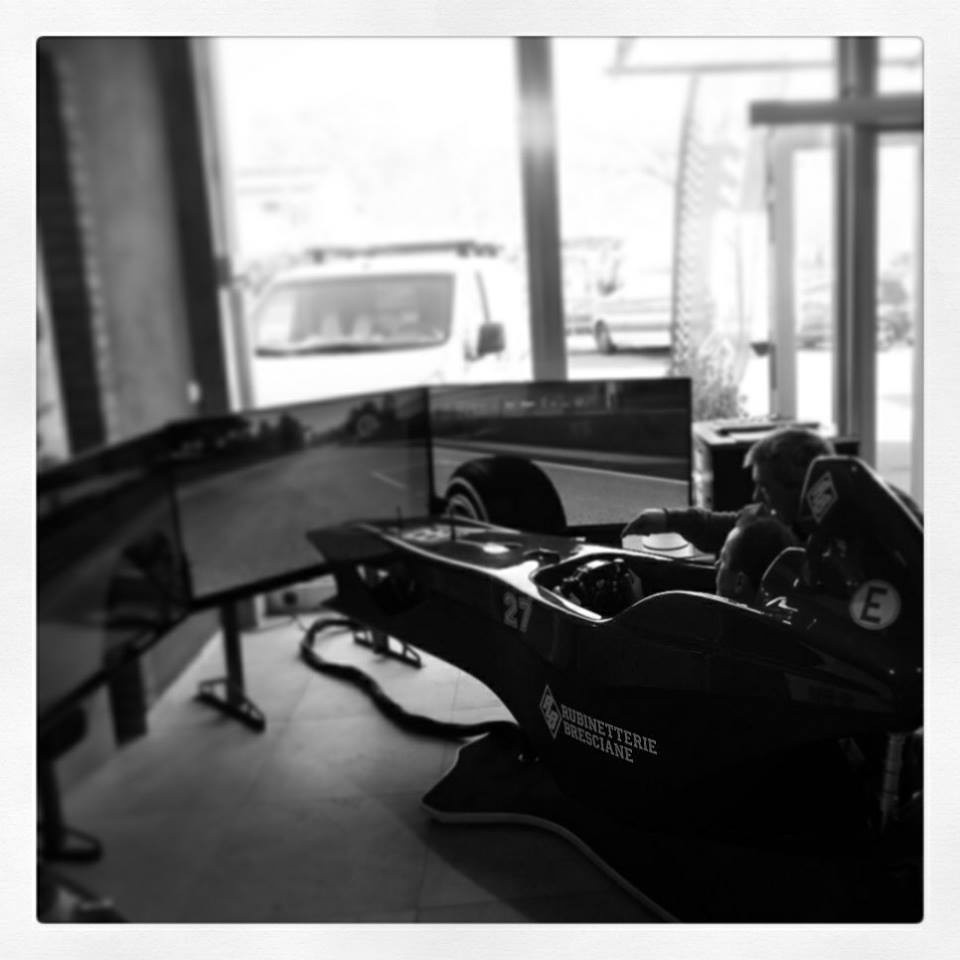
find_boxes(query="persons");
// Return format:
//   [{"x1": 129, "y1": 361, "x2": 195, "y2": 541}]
[
  {"x1": 625, "y1": 428, "x2": 924, "y2": 557},
  {"x1": 614, "y1": 515, "x2": 800, "y2": 609}
]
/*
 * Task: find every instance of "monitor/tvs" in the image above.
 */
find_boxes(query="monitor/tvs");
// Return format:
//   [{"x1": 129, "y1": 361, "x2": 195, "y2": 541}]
[{"x1": 164, "y1": 388, "x2": 434, "y2": 608}]
